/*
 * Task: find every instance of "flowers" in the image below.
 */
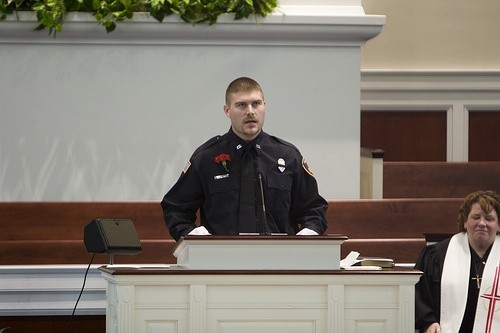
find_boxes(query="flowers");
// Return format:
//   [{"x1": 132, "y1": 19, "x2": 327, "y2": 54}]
[{"x1": 213, "y1": 153, "x2": 231, "y2": 173}]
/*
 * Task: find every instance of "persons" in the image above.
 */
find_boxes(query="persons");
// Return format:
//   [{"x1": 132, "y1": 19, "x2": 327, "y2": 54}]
[
  {"x1": 160, "y1": 77, "x2": 328, "y2": 242},
  {"x1": 412, "y1": 191, "x2": 500, "y2": 333}
]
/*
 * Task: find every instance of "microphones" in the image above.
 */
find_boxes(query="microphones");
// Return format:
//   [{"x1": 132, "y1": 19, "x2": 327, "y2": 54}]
[{"x1": 258, "y1": 173, "x2": 271, "y2": 236}]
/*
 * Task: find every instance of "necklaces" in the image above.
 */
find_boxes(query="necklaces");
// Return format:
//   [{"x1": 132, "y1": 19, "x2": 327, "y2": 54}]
[{"x1": 471, "y1": 252, "x2": 484, "y2": 288}]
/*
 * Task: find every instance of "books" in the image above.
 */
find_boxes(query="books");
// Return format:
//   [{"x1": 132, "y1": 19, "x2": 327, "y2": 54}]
[{"x1": 356, "y1": 257, "x2": 394, "y2": 268}]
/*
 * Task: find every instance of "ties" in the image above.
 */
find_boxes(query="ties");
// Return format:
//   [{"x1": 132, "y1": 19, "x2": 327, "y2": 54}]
[{"x1": 238, "y1": 141, "x2": 257, "y2": 233}]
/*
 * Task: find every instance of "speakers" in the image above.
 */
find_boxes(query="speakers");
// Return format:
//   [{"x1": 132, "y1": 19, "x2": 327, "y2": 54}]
[{"x1": 83, "y1": 217, "x2": 143, "y2": 255}]
